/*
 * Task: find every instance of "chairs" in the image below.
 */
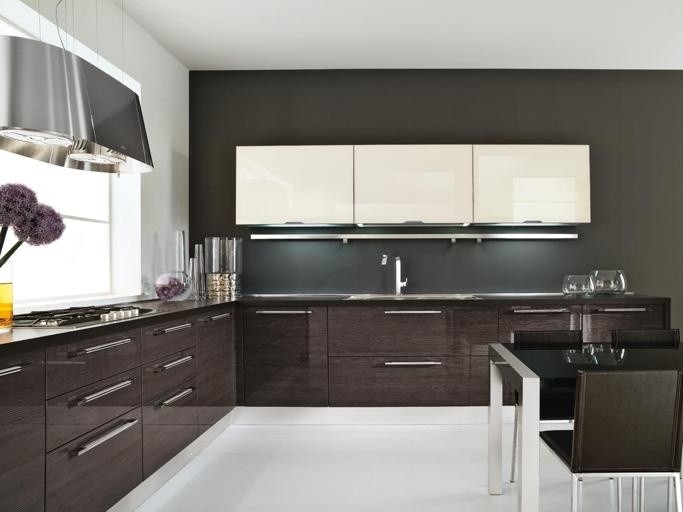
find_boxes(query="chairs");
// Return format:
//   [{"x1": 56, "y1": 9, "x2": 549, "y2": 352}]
[
  {"x1": 509, "y1": 330, "x2": 581, "y2": 480},
  {"x1": 539, "y1": 369, "x2": 678, "y2": 512},
  {"x1": 613, "y1": 329, "x2": 679, "y2": 349}
]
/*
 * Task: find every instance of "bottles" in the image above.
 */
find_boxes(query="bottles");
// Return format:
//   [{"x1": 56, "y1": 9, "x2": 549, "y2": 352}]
[
  {"x1": 153, "y1": 271, "x2": 195, "y2": 305},
  {"x1": 561, "y1": 267, "x2": 626, "y2": 303}
]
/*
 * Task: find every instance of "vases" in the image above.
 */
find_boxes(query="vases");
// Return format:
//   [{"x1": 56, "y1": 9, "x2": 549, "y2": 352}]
[
  {"x1": 0, "y1": 283, "x2": 13, "y2": 334},
  {"x1": 154, "y1": 272, "x2": 193, "y2": 302}
]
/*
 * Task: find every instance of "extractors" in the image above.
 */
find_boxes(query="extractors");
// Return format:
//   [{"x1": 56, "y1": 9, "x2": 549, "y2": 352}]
[{"x1": 0, "y1": 34, "x2": 153, "y2": 180}]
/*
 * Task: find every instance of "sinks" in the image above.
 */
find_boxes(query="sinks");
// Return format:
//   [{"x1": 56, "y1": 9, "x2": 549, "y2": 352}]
[{"x1": 343, "y1": 294, "x2": 482, "y2": 301}]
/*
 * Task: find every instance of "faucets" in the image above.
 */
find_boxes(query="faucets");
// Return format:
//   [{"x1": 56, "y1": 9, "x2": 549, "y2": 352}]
[{"x1": 380, "y1": 253, "x2": 403, "y2": 294}]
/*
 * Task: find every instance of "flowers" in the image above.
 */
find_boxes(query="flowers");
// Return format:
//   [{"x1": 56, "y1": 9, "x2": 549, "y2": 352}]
[{"x1": 0, "y1": 184, "x2": 65, "y2": 271}]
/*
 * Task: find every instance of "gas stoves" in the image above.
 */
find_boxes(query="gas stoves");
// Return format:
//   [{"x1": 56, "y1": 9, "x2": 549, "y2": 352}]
[{"x1": 11, "y1": 302, "x2": 153, "y2": 333}]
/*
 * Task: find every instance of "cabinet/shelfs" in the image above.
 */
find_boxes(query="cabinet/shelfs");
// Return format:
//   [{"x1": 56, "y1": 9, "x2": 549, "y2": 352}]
[
  {"x1": 116, "y1": 303, "x2": 196, "y2": 486},
  {"x1": 177, "y1": 283, "x2": 236, "y2": 445},
  {"x1": 327, "y1": 301, "x2": 498, "y2": 406},
  {"x1": 499, "y1": 293, "x2": 581, "y2": 344},
  {"x1": 353, "y1": 144, "x2": 472, "y2": 225},
  {"x1": 582, "y1": 294, "x2": 670, "y2": 344},
  {"x1": 472, "y1": 144, "x2": 591, "y2": 226},
  {"x1": 0, "y1": 323, "x2": 44, "y2": 511},
  {"x1": 239, "y1": 302, "x2": 325, "y2": 410},
  {"x1": 234, "y1": 144, "x2": 352, "y2": 227},
  {"x1": 6, "y1": 315, "x2": 141, "y2": 512}
]
